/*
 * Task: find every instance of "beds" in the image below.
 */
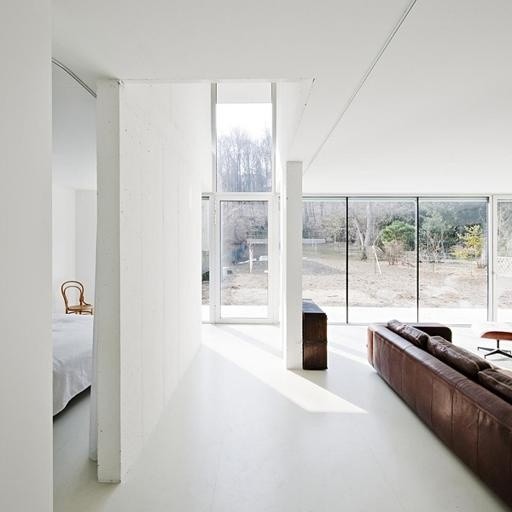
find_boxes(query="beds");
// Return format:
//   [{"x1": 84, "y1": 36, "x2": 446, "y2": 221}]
[{"x1": 52, "y1": 314, "x2": 93, "y2": 416}]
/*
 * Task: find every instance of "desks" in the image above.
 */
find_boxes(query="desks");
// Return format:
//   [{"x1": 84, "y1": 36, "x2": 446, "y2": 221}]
[{"x1": 473, "y1": 322, "x2": 512, "y2": 359}]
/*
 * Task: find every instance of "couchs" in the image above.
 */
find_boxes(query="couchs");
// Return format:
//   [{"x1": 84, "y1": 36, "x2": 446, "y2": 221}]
[{"x1": 367, "y1": 323, "x2": 512, "y2": 508}]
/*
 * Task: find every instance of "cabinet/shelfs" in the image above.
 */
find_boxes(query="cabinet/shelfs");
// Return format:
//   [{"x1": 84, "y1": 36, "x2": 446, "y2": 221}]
[{"x1": 303, "y1": 299, "x2": 327, "y2": 370}]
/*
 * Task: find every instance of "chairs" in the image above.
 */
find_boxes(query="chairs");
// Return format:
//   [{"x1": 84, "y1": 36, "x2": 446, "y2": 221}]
[{"x1": 61, "y1": 281, "x2": 93, "y2": 315}]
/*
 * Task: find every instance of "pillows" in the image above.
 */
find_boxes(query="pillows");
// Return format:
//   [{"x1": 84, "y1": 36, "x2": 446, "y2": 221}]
[{"x1": 388, "y1": 319, "x2": 512, "y2": 404}]
